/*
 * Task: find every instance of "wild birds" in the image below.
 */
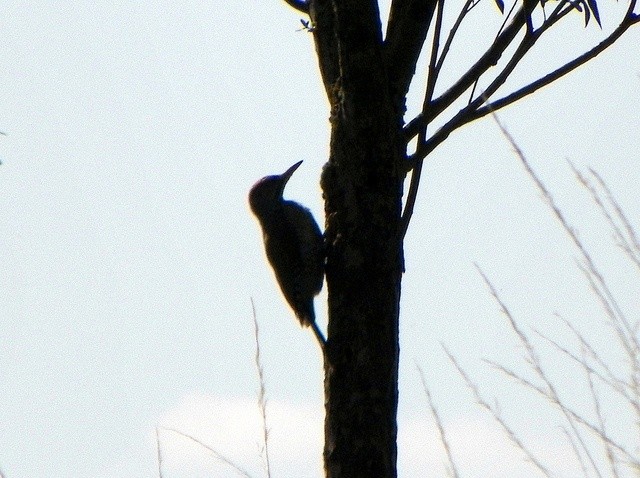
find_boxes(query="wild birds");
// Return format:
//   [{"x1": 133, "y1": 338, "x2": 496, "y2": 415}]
[{"x1": 248, "y1": 159, "x2": 340, "y2": 353}]
